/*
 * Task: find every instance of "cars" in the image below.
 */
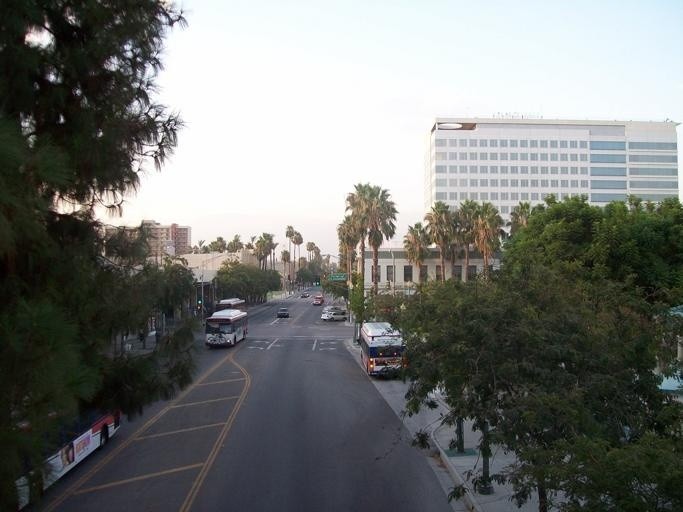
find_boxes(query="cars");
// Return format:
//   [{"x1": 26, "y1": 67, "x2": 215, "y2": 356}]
[
  {"x1": 313, "y1": 296, "x2": 323, "y2": 305},
  {"x1": 301, "y1": 293, "x2": 309, "y2": 298},
  {"x1": 277, "y1": 308, "x2": 289, "y2": 318},
  {"x1": 321, "y1": 307, "x2": 347, "y2": 321}
]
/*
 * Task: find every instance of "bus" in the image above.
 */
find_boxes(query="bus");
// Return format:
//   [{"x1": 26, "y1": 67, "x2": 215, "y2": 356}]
[
  {"x1": 216, "y1": 299, "x2": 246, "y2": 311},
  {"x1": 4, "y1": 400, "x2": 121, "y2": 512},
  {"x1": 357, "y1": 322, "x2": 407, "y2": 378},
  {"x1": 203, "y1": 309, "x2": 248, "y2": 346}
]
